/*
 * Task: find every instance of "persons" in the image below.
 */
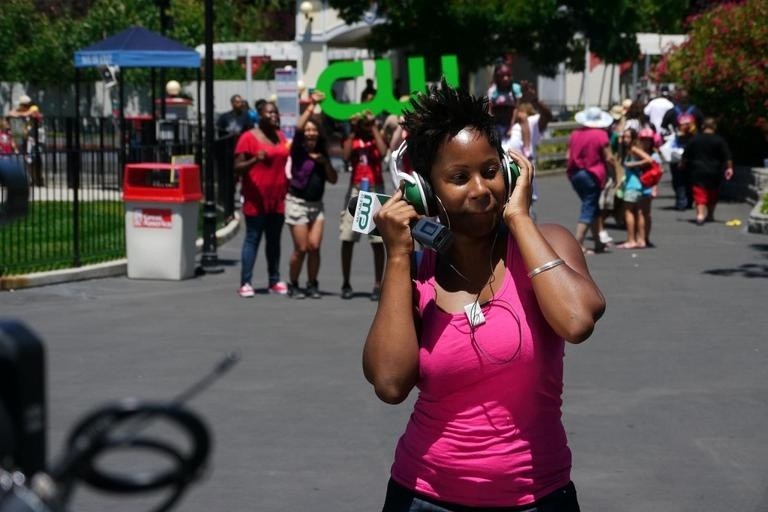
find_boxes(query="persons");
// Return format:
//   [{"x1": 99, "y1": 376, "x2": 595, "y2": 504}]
[
  {"x1": 216, "y1": 80, "x2": 416, "y2": 302},
  {"x1": 0, "y1": 118, "x2": 20, "y2": 185},
  {"x1": 662, "y1": 89, "x2": 703, "y2": 210},
  {"x1": 361, "y1": 79, "x2": 605, "y2": 511},
  {"x1": 679, "y1": 115, "x2": 733, "y2": 225},
  {"x1": 599, "y1": 86, "x2": 674, "y2": 248},
  {"x1": 10, "y1": 94, "x2": 45, "y2": 187},
  {"x1": 500, "y1": 79, "x2": 551, "y2": 227},
  {"x1": 566, "y1": 105, "x2": 614, "y2": 255},
  {"x1": 488, "y1": 64, "x2": 523, "y2": 161},
  {"x1": 93, "y1": 68, "x2": 117, "y2": 149}
]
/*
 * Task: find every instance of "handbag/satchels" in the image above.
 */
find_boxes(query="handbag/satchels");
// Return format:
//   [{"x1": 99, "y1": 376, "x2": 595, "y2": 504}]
[{"x1": 639, "y1": 159, "x2": 665, "y2": 190}]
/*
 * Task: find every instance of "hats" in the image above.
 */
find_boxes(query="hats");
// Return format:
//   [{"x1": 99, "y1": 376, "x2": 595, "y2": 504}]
[
  {"x1": 490, "y1": 94, "x2": 516, "y2": 110},
  {"x1": 14, "y1": 95, "x2": 33, "y2": 105},
  {"x1": 574, "y1": 104, "x2": 614, "y2": 130},
  {"x1": 608, "y1": 105, "x2": 628, "y2": 121}
]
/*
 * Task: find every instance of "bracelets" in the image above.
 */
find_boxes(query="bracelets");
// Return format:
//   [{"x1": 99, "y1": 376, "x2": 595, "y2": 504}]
[{"x1": 527, "y1": 257, "x2": 565, "y2": 278}]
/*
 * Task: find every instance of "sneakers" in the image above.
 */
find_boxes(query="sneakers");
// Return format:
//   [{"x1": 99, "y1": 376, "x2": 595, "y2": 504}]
[
  {"x1": 341, "y1": 285, "x2": 353, "y2": 299},
  {"x1": 370, "y1": 285, "x2": 381, "y2": 301},
  {"x1": 268, "y1": 280, "x2": 289, "y2": 296},
  {"x1": 236, "y1": 284, "x2": 255, "y2": 298},
  {"x1": 287, "y1": 280, "x2": 300, "y2": 297},
  {"x1": 305, "y1": 281, "x2": 322, "y2": 299}
]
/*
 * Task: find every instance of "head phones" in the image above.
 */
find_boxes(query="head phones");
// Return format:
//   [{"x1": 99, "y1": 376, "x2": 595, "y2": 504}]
[{"x1": 382, "y1": 135, "x2": 530, "y2": 220}]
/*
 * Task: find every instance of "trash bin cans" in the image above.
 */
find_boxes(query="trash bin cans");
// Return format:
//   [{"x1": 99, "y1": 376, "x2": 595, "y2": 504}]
[{"x1": 122, "y1": 161, "x2": 205, "y2": 281}]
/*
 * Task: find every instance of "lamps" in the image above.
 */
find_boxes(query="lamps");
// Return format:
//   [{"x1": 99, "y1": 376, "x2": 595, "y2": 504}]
[
  {"x1": 165, "y1": 79, "x2": 182, "y2": 97},
  {"x1": 300, "y1": 2, "x2": 313, "y2": 22}
]
